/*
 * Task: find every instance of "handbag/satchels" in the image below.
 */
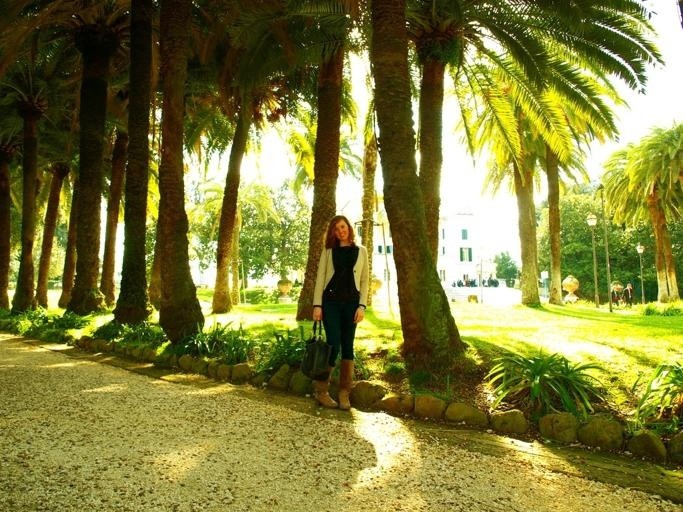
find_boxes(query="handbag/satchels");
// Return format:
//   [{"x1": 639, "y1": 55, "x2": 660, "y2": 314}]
[{"x1": 301, "y1": 338, "x2": 334, "y2": 381}]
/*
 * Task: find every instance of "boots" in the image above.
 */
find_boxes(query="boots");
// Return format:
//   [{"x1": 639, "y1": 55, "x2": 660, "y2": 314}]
[
  {"x1": 337, "y1": 359, "x2": 354, "y2": 411},
  {"x1": 314, "y1": 365, "x2": 338, "y2": 408}
]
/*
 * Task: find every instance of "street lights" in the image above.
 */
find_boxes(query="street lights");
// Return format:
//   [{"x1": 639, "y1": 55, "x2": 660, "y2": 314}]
[
  {"x1": 636, "y1": 242, "x2": 646, "y2": 305},
  {"x1": 586, "y1": 212, "x2": 599, "y2": 308}
]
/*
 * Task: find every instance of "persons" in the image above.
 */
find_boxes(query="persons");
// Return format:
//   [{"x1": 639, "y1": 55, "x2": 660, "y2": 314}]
[
  {"x1": 612, "y1": 282, "x2": 634, "y2": 309},
  {"x1": 311, "y1": 215, "x2": 370, "y2": 410},
  {"x1": 451, "y1": 274, "x2": 499, "y2": 287}
]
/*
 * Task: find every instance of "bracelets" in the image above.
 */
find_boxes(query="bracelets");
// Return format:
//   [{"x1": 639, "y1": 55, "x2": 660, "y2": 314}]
[{"x1": 360, "y1": 305, "x2": 366, "y2": 311}]
